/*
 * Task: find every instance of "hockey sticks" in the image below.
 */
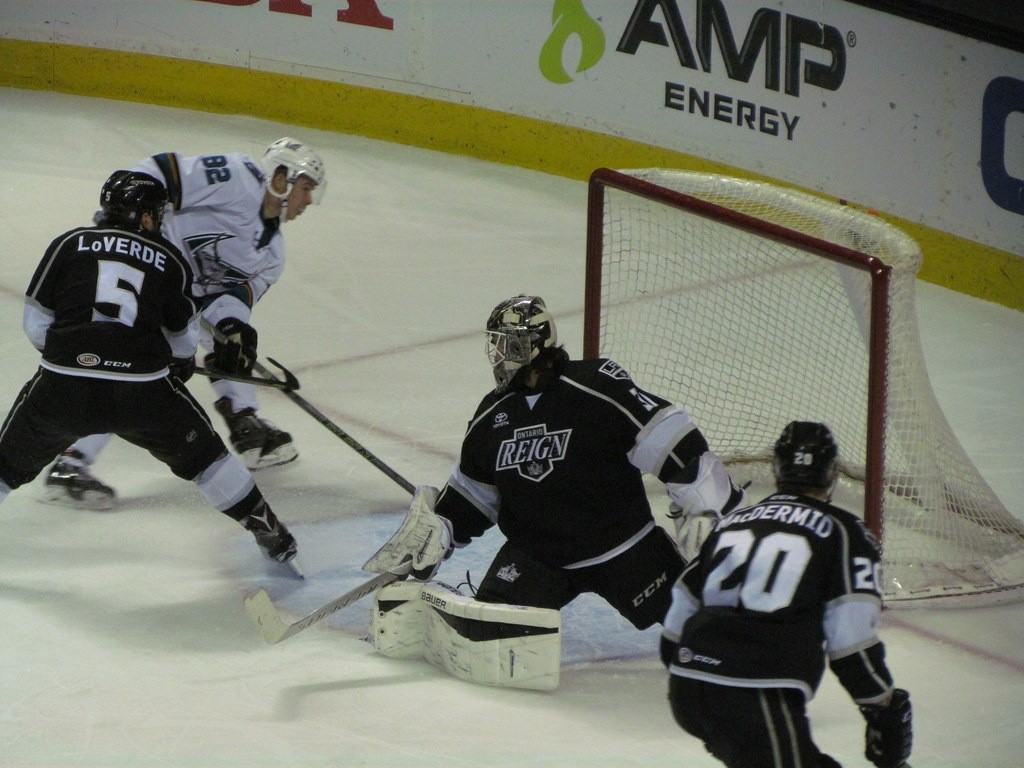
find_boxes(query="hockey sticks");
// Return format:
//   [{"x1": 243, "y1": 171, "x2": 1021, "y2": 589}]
[
  {"x1": 199, "y1": 317, "x2": 419, "y2": 497},
  {"x1": 242, "y1": 560, "x2": 412, "y2": 647},
  {"x1": 166, "y1": 358, "x2": 300, "y2": 391}
]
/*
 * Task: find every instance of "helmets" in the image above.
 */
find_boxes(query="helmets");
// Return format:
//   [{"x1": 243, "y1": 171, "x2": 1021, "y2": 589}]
[
  {"x1": 261, "y1": 136, "x2": 324, "y2": 201},
  {"x1": 774, "y1": 421, "x2": 838, "y2": 492},
  {"x1": 484, "y1": 295, "x2": 557, "y2": 393},
  {"x1": 100, "y1": 170, "x2": 166, "y2": 210}
]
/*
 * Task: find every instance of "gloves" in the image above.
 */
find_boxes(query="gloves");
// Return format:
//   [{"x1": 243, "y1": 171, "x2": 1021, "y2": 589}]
[
  {"x1": 213, "y1": 316, "x2": 259, "y2": 374},
  {"x1": 171, "y1": 350, "x2": 196, "y2": 383},
  {"x1": 859, "y1": 688, "x2": 913, "y2": 768}
]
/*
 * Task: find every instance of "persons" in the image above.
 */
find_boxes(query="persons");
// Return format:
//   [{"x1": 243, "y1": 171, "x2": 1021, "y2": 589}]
[
  {"x1": 0, "y1": 137, "x2": 325, "y2": 581},
  {"x1": 363, "y1": 293, "x2": 754, "y2": 693},
  {"x1": 658, "y1": 421, "x2": 913, "y2": 768}
]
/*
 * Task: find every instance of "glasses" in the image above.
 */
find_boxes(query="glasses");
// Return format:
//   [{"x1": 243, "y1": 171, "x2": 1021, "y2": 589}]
[
  {"x1": 308, "y1": 178, "x2": 328, "y2": 205},
  {"x1": 159, "y1": 200, "x2": 174, "y2": 224}
]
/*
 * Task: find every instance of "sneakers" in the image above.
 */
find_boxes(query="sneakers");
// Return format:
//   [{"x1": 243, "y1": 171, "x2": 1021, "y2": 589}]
[
  {"x1": 215, "y1": 396, "x2": 300, "y2": 470},
  {"x1": 36, "y1": 449, "x2": 115, "y2": 510},
  {"x1": 246, "y1": 504, "x2": 305, "y2": 579}
]
